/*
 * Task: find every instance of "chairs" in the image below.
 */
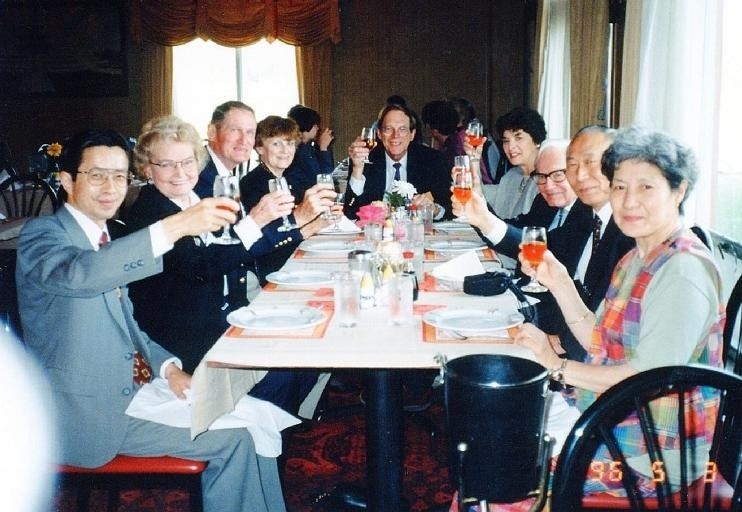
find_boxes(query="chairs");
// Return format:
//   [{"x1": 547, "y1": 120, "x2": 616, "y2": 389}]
[
  {"x1": 0, "y1": 243, "x2": 204, "y2": 512},
  {"x1": 0, "y1": 172, "x2": 58, "y2": 218},
  {"x1": 551, "y1": 363, "x2": 740, "y2": 512},
  {"x1": 710, "y1": 229, "x2": 742, "y2": 375}
]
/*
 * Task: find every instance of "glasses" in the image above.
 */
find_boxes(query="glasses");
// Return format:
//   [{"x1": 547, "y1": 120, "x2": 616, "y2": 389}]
[
  {"x1": 381, "y1": 125, "x2": 411, "y2": 134},
  {"x1": 147, "y1": 156, "x2": 200, "y2": 169},
  {"x1": 76, "y1": 167, "x2": 129, "y2": 187},
  {"x1": 529, "y1": 168, "x2": 566, "y2": 185}
]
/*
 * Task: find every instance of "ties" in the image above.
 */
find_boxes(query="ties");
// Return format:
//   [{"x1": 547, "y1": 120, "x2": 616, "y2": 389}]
[
  {"x1": 98, "y1": 231, "x2": 154, "y2": 383},
  {"x1": 557, "y1": 208, "x2": 564, "y2": 227},
  {"x1": 393, "y1": 163, "x2": 402, "y2": 181},
  {"x1": 590, "y1": 214, "x2": 602, "y2": 258}
]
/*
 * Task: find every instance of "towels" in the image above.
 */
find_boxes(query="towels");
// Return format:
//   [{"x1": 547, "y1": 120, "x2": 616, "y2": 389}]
[{"x1": 123, "y1": 374, "x2": 303, "y2": 463}]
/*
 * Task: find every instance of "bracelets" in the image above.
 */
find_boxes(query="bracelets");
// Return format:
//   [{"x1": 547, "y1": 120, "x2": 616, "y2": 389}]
[{"x1": 548, "y1": 356, "x2": 567, "y2": 386}]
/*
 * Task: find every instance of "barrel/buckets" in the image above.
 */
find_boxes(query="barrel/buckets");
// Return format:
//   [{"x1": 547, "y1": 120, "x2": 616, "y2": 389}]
[{"x1": 432, "y1": 354, "x2": 565, "y2": 506}]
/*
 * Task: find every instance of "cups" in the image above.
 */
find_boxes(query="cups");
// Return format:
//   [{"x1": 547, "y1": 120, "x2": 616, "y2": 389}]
[
  {"x1": 392, "y1": 211, "x2": 409, "y2": 244},
  {"x1": 314, "y1": 226, "x2": 364, "y2": 235},
  {"x1": 385, "y1": 273, "x2": 413, "y2": 328},
  {"x1": 453, "y1": 169, "x2": 473, "y2": 223},
  {"x1": 416, "y1": 201, "x2": 435, "y2": 236},
  {"x1": 422, "y1": 239, "x2": 489, "y2": 254},
  {"x1": 521, "y1": 226, "x2": 550, "y2": 293},
  {"x1": 268, "y1": 176, "x2": 300, "y2": 234},
  {"x1": 409, "y1": 218, "x2": 425, "y2": 245},
  {"x1": 465, "y1": 122, "x2": 484, "y2": 161},
  {"x1": 211, "y1": 176, "x2": 240, "y2": 245},
  {"x1": 334, "y1": 272, "x2": 363, "y2": 328},
  {"x1": 400, "y1": 239, "x2": 416, "y2": 261},
  {"x1": 315, "y1": 173, "x2": 337, "y2": 221},
  {"x1": 360, "y1": 127, "x2": 376, "y2": 164},
  {"x1": 363, "y1": 224, "x2": 383, "y2": 251}
]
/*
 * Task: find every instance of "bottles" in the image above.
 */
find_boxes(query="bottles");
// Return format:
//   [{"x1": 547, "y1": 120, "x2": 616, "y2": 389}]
[
  {"x1": 401, "y1": 252, "x2": 418, "y2": 301},
  {"x1": 406, "y1": 206, "x2": 424, "y2": 281}
]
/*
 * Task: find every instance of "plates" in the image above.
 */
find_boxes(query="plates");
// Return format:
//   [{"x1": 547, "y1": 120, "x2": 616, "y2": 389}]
[
  {"x1": 428, "y1": 266, "x2": 511, "y2": 284},
  {"x1": 225, "y1": 303, "x2": 328, "y2": 332},
  {"x1": 433, "y1": 223, "x2": 472, "y2": 231},
  {"x1": 265, "y1": 269, "x2": 341, "y2": 286},
  {"x1": 421, "y1": 302, "x2": 525, "y2": 332},
  {"x1": 298, "y1": 238, "x2": 359, "y2": 257}
]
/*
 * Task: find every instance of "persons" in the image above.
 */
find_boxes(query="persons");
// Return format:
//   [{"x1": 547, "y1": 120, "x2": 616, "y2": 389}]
[
  {"x1": 368, "y1": 97, "x2": 420, "y2": 146},
  {"x1": 16, "y1": 129, "x2": 284, "y2": 511},
  {"x1": 420, "y1": 101, "x2": 492, "y2": 185},
  {"x1": 450, "y1": 139, "x2": 579, "y2": 305},
  {"x1": 450, "y1": 129, "x2": 725, "y2": 512},
  {"x1": 123, "y1": 116, "x2": 294, "y2": 470},
  {"x1": 450, "y1": 97, "x2": 501, "y2": 184},
  {"x1": 193, "y1": 102, "x2": 337, "y2": 402},
  {"x1": 343, "y1": 103, "x2": 458, "y2": 223},
  {"x1": 283, "y1": 104, "x2": 334, "y2": 206},
  {"x1": 239, "y1": 116, "x2": 344, "y2": 284},
  {"x1": 450, "y1": 126, "x2": 636, "y2": 394},
  {"x1": 463, "y1": 107, "x2": 549, "y2": 218}
]
